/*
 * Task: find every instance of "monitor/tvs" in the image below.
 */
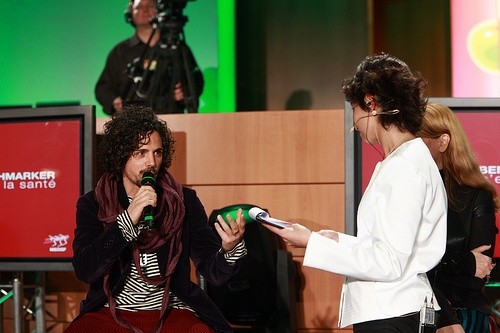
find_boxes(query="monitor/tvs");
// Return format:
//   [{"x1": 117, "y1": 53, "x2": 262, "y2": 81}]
[{"x1": 0, "y1": 106, "x2": 97, "y2": 272}]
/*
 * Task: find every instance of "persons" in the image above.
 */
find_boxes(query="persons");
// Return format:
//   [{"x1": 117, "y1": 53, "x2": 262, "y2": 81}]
[
  {"x1": 94, "y1": 0, "x2": 205, "y2": 115},
  {"x1": 416, "y1": 102, "x2": 500, "y2": 333},
  {"x1": 259, "y1": 51, "x2": 448, "y2": 333},
  {"x1": 63, "y1": 105, "x2": 247, "y2": 333}
]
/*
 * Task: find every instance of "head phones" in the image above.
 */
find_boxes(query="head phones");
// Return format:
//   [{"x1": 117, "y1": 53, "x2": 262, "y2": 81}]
[{"x1": 126, "y1": 11, "x2": 132, "y2": 23}]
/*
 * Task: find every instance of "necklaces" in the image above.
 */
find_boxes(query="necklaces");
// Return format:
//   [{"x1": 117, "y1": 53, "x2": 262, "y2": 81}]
[{"x1": 386, "y1": 132, "x2": 410, "y2": 157}]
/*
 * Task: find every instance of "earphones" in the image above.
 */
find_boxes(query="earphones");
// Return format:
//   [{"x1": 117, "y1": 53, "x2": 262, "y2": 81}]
[{"x1": 367, "y1": 102, "x2": 371, "y2": 106}]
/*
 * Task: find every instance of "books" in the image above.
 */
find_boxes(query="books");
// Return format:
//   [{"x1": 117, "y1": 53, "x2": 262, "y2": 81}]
[{"x1": 248, "y1": 207, "x2": 294, "y2": 232}]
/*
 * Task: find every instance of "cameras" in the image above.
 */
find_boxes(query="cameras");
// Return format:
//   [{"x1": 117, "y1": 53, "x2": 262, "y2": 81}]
[{"x1": 148, "y1": 0, "x2": 188, "y2": 45}]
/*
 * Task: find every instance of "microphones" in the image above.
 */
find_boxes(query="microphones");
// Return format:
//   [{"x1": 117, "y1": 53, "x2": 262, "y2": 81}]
[
  {"x1": 141, "y1": 171, "x2": 156, "y2": 228},
  {"x1": 350, "y1": 109, "x2": 399, "y2": 132}
]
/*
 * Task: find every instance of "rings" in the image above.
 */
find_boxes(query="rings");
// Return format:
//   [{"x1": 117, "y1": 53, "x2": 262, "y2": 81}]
[
  {"x1": 491, "y1": 258, "x2": 496, "y2": 264},
  {"x1": 233, "y1": 231, "x2": 238, "y2": 235}
]
